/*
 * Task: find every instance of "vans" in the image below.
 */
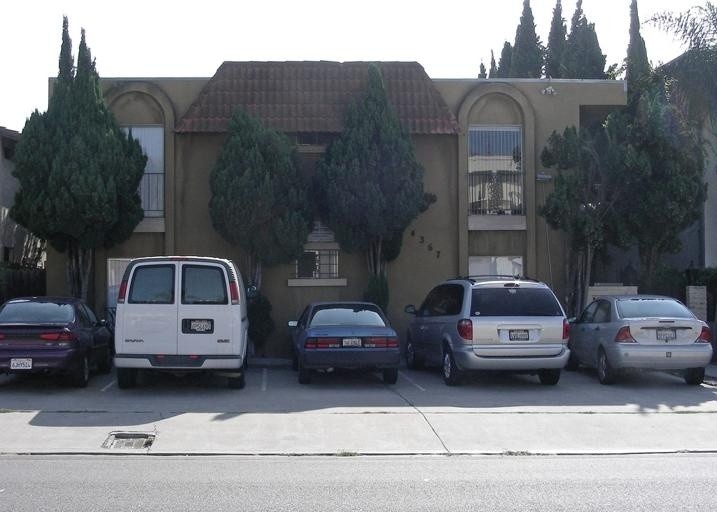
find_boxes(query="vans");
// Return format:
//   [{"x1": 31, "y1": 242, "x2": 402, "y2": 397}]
[
  {"x1": 115, "y1": 255, "x2": 258, "y2": 390},
  {"x1": 405, "y1": 274, "x2": 571, "y2": 386}
]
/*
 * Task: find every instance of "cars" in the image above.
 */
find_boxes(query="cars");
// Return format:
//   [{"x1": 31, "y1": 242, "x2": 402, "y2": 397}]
[
  {"x1": 570, "y1": 294, "x2": 713, "y2": 385},
  {"x1": 288, "y1": 300, "x2": 401, "y2": 385},
  {"x1": 1, "y1": 295, "x2": 114, "y2": 388}
]
